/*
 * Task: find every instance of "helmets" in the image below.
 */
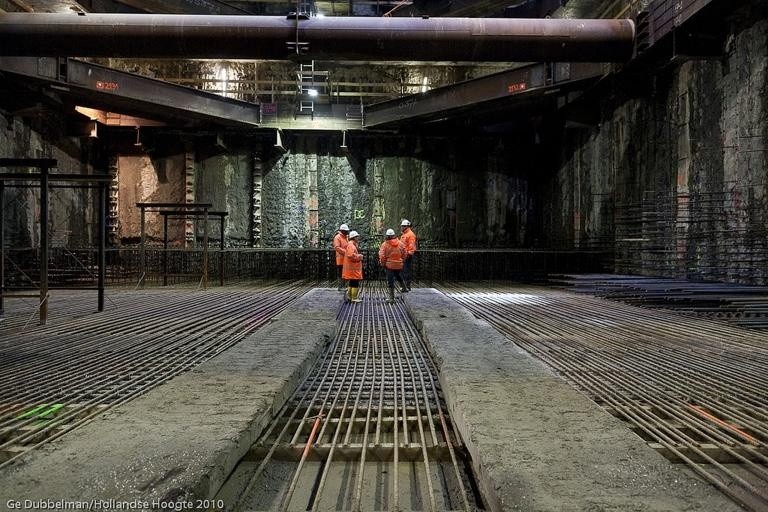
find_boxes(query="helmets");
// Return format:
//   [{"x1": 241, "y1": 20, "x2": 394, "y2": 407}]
[
  {"x1": 386, "y1": 228, "x2": 395, "y2": 236},
  {"x1": 349, "y1": 231, "x2": 359, "y2": 238},
  {"x1": 401, "y1": 220, "x2": 411, "y2": 225},
  {"x1": 339, "y1": 224, "x2": 350, "y2": 230}
]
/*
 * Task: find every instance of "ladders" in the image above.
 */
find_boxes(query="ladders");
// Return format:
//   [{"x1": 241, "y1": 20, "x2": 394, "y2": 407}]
[
  {"x1": 299, "y1": 60, "x2": 314, "y2": 113},
  {"x1": 341, "y1": 80, "x2": 364, "y2": 148}
]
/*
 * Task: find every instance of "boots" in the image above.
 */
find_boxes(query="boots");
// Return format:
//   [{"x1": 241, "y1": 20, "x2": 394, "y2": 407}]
[
  {"x1": 338, "y1": 277, "x2": 348, "y2": 291},
  {"x1": 385, "y1": 280, "x2": 409, "y2": 303},
  {"x1": 347, "y1": 287, "x2": 363, "y2": 302}
]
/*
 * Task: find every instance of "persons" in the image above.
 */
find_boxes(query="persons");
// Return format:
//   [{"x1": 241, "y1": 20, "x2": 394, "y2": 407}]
[
  {"x1": 399, "y1": 218, "x2": 416, "y2": 291},
  {"x1": 377, "y1": 228, "x2": 409, "y2": 303},
  {"x1": 342, "y1": 230, "x2": 368, "y2": 303},
  {"x1": 333, "y1": 223, "x2": 351, "y2": 290}
]
